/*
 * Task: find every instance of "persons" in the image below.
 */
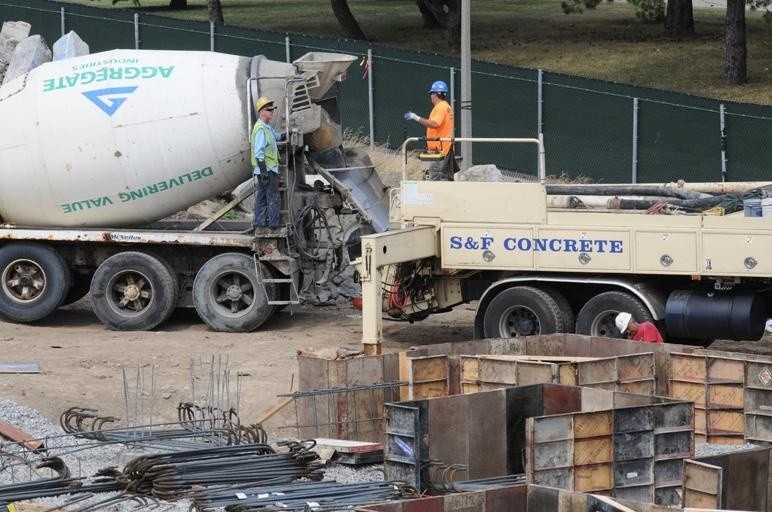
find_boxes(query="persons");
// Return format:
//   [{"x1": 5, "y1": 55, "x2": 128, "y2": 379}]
[
  {"x1": 614, "y1": 312, "x2": 664, "y2": 345},
  {"x1": 250, "y1": 96, "x2": 286, "y2": 229},
  {"x1": 404, "y1": 80, "x2": 460, "y2": 180}
]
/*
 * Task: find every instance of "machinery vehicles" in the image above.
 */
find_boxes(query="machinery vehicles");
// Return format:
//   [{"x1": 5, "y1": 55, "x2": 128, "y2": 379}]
[{"x1": 1, "y1": 19, "x2": 389, "y2": 333}]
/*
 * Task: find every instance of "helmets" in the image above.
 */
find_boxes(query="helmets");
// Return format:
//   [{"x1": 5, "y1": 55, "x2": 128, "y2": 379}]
[
  {"x1": 427, "y1": 79, "x2": 449, "y2": 96},
  {"x1": 615, "y1": 311, "x2": 632, "y2": 334},
  {"x1": 255, "y1": 97, "x2": 275, "y2": 113}
]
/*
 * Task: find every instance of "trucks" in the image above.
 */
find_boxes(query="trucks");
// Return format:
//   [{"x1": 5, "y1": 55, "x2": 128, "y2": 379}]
[{"x1": 360, "y1": 136, "x2": 772, "y2": 355}]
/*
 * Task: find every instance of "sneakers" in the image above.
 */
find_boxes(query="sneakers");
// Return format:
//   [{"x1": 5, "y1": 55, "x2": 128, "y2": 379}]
[{"x1": 255, "y1": 226, "x2": 288, "y2": 238}]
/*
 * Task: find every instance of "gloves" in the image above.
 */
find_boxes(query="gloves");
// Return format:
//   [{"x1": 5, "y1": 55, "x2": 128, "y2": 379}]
[
  {"x1": 281, "y1": 131, "x2": 291, "y2": 141},
  {"x1": 257, "y1": 161, "x2": 270, "y2": 189},
  {"x1": 404, "y1": 110, "x2": 421, "y2": 122}
]
